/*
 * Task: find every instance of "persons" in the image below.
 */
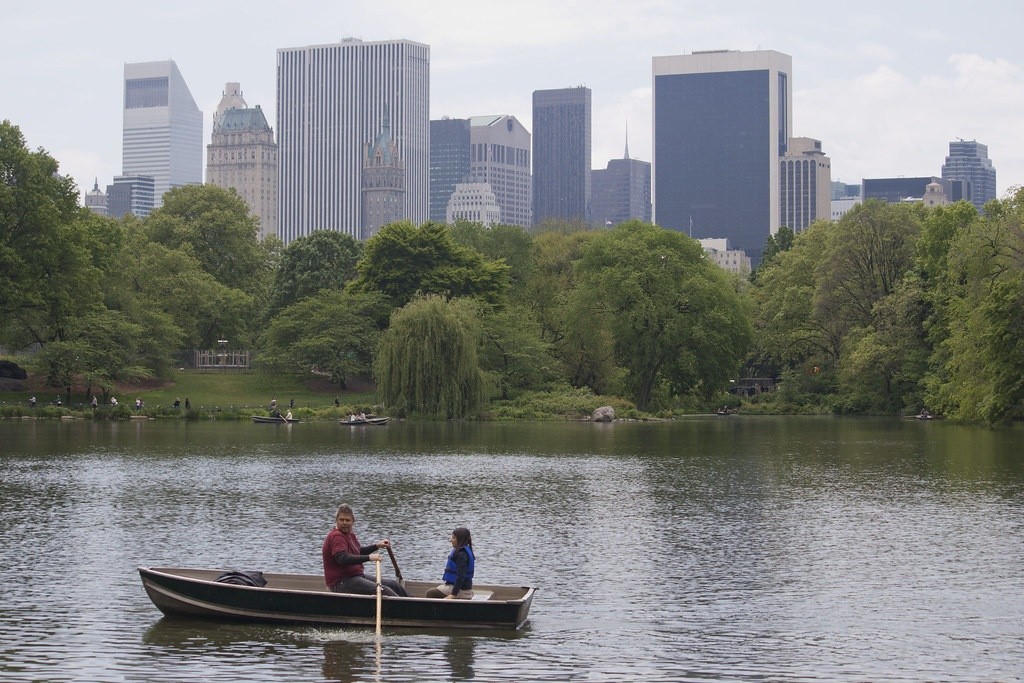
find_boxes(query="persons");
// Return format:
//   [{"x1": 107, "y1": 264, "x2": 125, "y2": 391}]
[
  {"x1": 285, "y1": 410, "x2": 292, "y2": 420},
  {"x1": 174, "y1": 398, "x2": 180, "y2": 410},
  {"x1": 426, "y1": 528, "x2": 476, "y2": 599},
  {"x1": 29, "y1": 395, "x2": 36, "y2": 407},
  {"x1": 93, "y1": 396, "x2": 97, "y2": 409},
  {"x1": 335, "y1": 397, "x2": 339, "y2": 407},
  {"x1": 110, "y1": 396, "x2": 118, "y2": 407},
  {"x1": 323, "y1": 505, "x2": 412, "y2": 597},
  {"x1": 54, "y1": 395, "x2": 62, "y2": 407},
  {"x1": 135, "y1": 397, "x2": 143, "y2": 411},
  {"x1": 185, "y1": 398, "x2": 191, "y2": 409},
  {"x1": 277, "y1": 409, "x2": 282, "y2": 418},
  {"x1": 349, "y1": 411, "x2": 366, "y2": 422},
  {"x1": 291, "y1": 399, "x2": 294, "y2": 409}
]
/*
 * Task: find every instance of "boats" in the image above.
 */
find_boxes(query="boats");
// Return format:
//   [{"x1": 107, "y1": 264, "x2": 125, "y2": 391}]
[
  {"x1": 717, "y1": 408, "x2": 731, "y2": 415},
  {"x1": 339, "y1": 417, "x2": 389, "y2": 426},
  {"x1": 916, "y1": 413, "x2": 934, "y2": 420},
  {"x1": 138, "y1": 565, "x2": 541, "y2": 630},
  {"x1": 251, "y1": 415, "x2": 301, "y2": 424}
]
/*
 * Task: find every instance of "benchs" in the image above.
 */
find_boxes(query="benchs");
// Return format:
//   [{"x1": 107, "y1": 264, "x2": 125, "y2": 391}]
[{"x1": 472, "y1": 591, "x2": 494, "y2": 600}]
